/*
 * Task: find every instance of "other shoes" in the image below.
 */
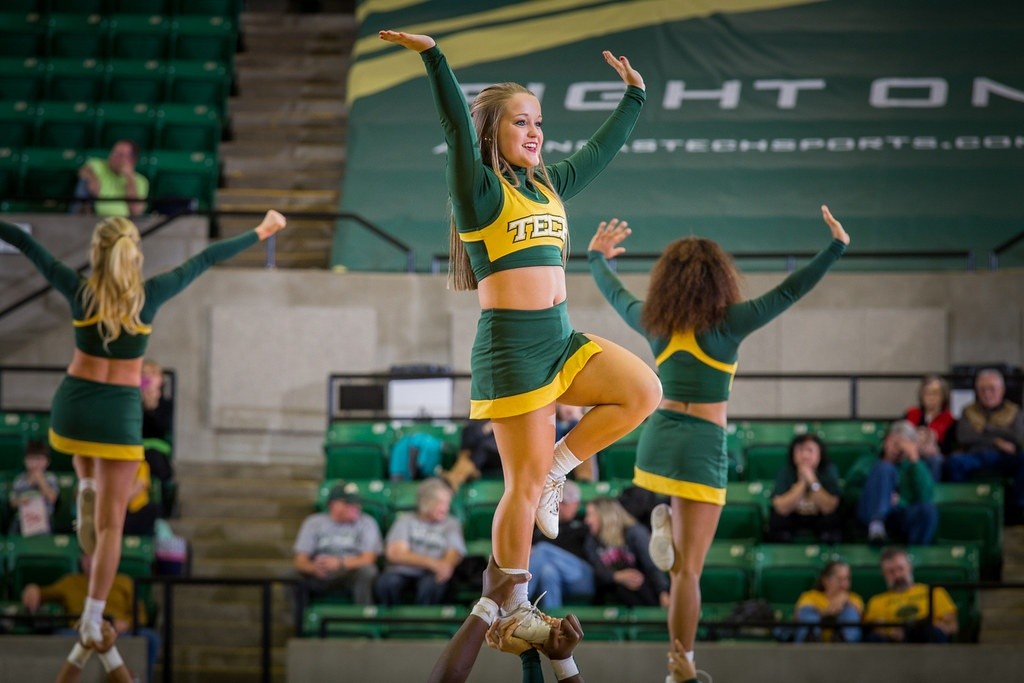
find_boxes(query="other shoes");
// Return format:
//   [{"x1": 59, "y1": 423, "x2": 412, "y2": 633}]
[
  {"x1": 77, "y1": 480, "x2": 97, "y2": 556},
  {"x1": 868, "y1": 534, "x2": 886, "y2": 546},
  {"x1": 79, "y1": 621, "x2": 103, "y2": 645},
  {"x1": 649, "y1": 504, "x2": 675, "y2": 571}
]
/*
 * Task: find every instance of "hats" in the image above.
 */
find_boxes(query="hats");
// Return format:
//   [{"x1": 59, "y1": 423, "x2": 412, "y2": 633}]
[
  {"x1": 25, "y1": 440, "x2": 48, "y2": 456},
  {"x1": 330, "y1": 482, "x2": 366, "y2": 503}
]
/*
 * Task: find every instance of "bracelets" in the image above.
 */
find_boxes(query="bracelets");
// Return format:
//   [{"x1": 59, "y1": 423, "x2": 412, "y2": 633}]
[
  {"x1": 550, "y1": 655, "x2": 579, "y2": 681},
  {"x1": 98, "y1": 644, "x2": 123, "y2": 675},
  {"x1": 470, "y1": 597, "x2": 499, "y2": 627},
  {"x1": 338, "y1": 555, "x2": 346, "y2": 570},
  {"x1": 65, "y1": 642, "x2": 93, "y2": 671}
]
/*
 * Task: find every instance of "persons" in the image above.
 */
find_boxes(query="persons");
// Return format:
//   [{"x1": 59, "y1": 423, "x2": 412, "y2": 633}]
[
  {"x1": 74, "y1": 141, "x2": 150, "y2": 219},
  {"x1": 519, "y1": 480, "x2": 596, "y2": 608},
  {"x1": 586, "y1": 498, "x2": 669, "y2": 607},
  {"x1": 54, "y1": 623, "x2": 134, "y2": 683},
  {"x1": 437, "y1": 403, "x2": 603, "y2": 490},
  {"x1": 789, "y1": 560, "x2": 864, "y2": 643},
  {"x1": 379, "y1": 478, "x2": 466, "y2": 609},
  {"x1": 587, "y1": 203, "x2": 851, "y2": 683},
  {"x1": 6, "y1": 353, "x2": 171, "y2": 642},
  {"x1": 378, "y1": 28, "x2": 665, "y2": 644},
  {"x1": 1, "y1": 207, "x2": 288, "y2": 643},
  {"x1": 430, "y1": 555, "x2": 587, "y2": 683},
  {"x1": 763, "y1": 434, "x2": 848, "y2": 545},
  {"x1": 289, "y1": 481, "x2": 381, "y2": 608},
  {"x1": 952, "y1": 370, "x2": 1019, "y2": 482},
  {"x1": 864, "y1": 548, "x2": 962, "y2": 644},
  {"x1": 847, "y1": 421, "x2": 941, "y2": 550},
  {"x1": 900, "y1": 375, "x2": 958, "y2": 483}
]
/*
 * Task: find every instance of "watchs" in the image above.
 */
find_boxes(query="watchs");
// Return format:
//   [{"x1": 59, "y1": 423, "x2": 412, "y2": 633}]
[{"x1": 810, "y1": 482, "x2": 822, "y2": 492}]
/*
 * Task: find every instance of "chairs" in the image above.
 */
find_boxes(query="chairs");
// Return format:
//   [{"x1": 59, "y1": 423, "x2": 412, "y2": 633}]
[
  {"x1": 0, "y1": 413, "x2": 159, "y2": 635},
  {"x1": 0, "y1": 0, "x2": 247, "y2": 241},
  {"x1": 303, "y1": 421, "x2": 1006, "y2": 646}
]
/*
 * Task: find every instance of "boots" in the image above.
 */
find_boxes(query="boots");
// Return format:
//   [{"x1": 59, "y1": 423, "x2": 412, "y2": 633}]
[{"x1": 434, "y1": 457, "x2": 476, "y2": 491}]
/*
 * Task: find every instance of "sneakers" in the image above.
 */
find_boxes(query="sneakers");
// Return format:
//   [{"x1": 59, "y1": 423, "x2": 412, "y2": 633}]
[
  {"x1": 492, "y1": 591, "x2": 566, "y2": 644},
  {"x1": 535, "y1": 475, "x2": 566, "y2": 539}
]
